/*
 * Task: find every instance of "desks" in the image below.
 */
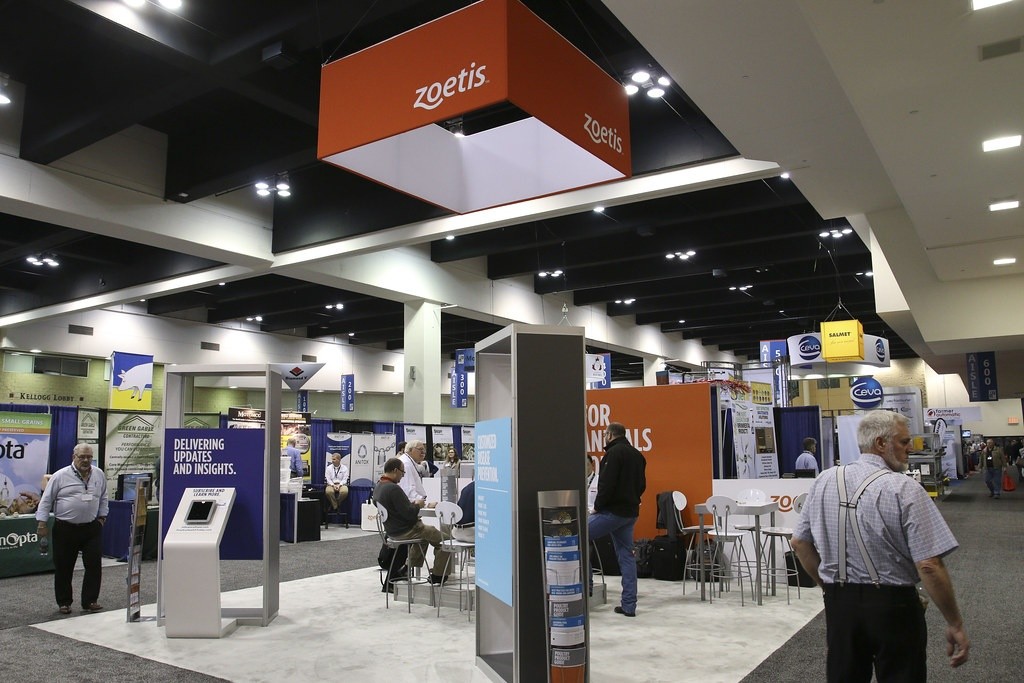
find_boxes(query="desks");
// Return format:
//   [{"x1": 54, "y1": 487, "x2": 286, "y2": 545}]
[
  {"x1": 297, "y1": 483, "x2": 323, "y2": 541},
  {"x1": 695, "y1": 500, "x2": 780, "y2": 606},
  {"x1": 0, "y1": 513, "x2": 56, "y2": 578},
  {"x1": 100, "y1": 500, "x2": 159, "y2": 562},
  {"x1": 415, "y1": 507, "x2": 437, "y2": 580}
]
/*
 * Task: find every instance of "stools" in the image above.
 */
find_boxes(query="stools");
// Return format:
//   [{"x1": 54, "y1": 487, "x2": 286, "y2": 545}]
[
  {"x1": 673, "y1": 490, "x2": 809, "y2": 605},
  {"x1": 323, "y1": 477, "x2": 351, "y2": 530},
  {"x1": 375, "y1": 500, "x2": 476, "y2": 623}
]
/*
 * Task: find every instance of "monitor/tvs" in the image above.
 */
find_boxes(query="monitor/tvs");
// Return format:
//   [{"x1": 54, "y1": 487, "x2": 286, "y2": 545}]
[
  {"x1": 184, "y1": 500, "x2": 216, "y2": 523},
  {"x1": 118, "y1": 473, "x2": 152, "y2": 501}
]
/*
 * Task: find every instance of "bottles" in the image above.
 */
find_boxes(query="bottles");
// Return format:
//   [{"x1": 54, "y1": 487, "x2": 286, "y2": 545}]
[{"x1": 40, "y1": 536, "x2": 50, "y2": 556}]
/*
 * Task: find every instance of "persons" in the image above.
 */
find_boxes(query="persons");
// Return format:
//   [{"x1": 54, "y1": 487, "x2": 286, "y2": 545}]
[
  {"x1": 1006, "y1": 439, "x2": 1023, "y2": 465},
  {"x1": 443, "y1": 448, "x2": 461, "y2": 477},
  {"x1": 373, "y1": 458, "x2": 451, "y2": 584},
  {"x1": 452, "y1": 481, "x2": 475, "y2": 542},
  {"x1": 325, "y1": 453, "x2": 349, "y2": 512},
  {"x1": 381, "y1": 441, "x2": 439, "y2": 593},
  {"x1": 589, "y1": 422, "x2": 647, "y2": 617},
  {"x1": 587, "y1": 455, "x2": 599, "y2": 513},
  {"x1": 286, "y1": 438, "x2": 303, "y2": 478},
  {"x1": 36, "y1": 443, "x2": 109, "y2": 613},
  {"x1": 795, "y1": 438, "x2": 819, "y2": 476},
  {"x1": 791, "y1": 410, "x2": 969, "y2": 683},
  {"x1": 978, "y1": 439, "x2": 1008, "y2": 498}
]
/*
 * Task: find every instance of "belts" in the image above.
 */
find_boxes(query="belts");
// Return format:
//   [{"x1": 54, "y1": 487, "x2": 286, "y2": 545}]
[{"x1": 454, "y1": 523, "x2": 475, "y2": 528}]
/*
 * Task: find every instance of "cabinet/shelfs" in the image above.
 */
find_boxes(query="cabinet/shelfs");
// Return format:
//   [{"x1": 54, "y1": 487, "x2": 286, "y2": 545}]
[{"x1": 907, "y1": 433, "x2": 945, "y2": 505}]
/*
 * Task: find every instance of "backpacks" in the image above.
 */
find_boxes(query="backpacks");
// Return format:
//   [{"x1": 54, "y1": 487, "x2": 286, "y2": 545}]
[
  {"x1": 649, "y1": 535, "x2": 686, "y2": 581},
  {"x1": 633, "y1": 539, "x2": 652, "y2": 578},
  {"x1": 692, "y1": 540, "x2": 720, "y2": 581},
  {"x1": 378, "y1": 533, "x2": 408, "y2": 569}
]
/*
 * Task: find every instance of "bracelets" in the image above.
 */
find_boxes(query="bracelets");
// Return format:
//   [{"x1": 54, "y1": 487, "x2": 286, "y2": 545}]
[
  {"x1": 37, "y1": 525, "x2": 47, "y2": 529},
  {"x1": 99, "y1": 517, "x2": 106, "y2": 522}
]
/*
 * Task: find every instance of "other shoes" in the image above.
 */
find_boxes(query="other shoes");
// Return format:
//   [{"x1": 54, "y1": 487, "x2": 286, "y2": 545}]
[
  {"x1": 59, "y1": 605, "x2": 71, "y2": 614},
  {"x1": 989, "y1": 490, "x2": 994, "y2": 496},
  {"x1": 427, "y1": 574, "x2": 448, "y2": 584},
  {"x1": 993, "y1": 493, "x2": 1000, "y2": 498},
  {"x1": 81, "y1": 602, "x2": 103, "y2": 612},
  {"x1": 615, "y1": 606, "x2": 635, "y2": 617}
]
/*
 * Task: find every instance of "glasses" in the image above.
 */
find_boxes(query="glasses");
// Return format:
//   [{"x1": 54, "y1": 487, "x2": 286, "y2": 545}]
[
  {"x1": 393, "y1": 467, "x2": 406, "y2": 474},
  {"x1": 75, "y1": 454, "x2": 92, "y2": 461},
  {"x1": 602, "y1": 433, "x2": 614, "y2": 437}
]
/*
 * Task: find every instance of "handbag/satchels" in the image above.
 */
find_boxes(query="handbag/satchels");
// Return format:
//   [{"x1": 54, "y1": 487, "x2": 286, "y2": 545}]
[
  {"x1": 1003, "y1": 471, "x2": 1016, "y2": 491},
  {"x1": 592, "y1": 534, "x2": 620, "y2": 575}
]
